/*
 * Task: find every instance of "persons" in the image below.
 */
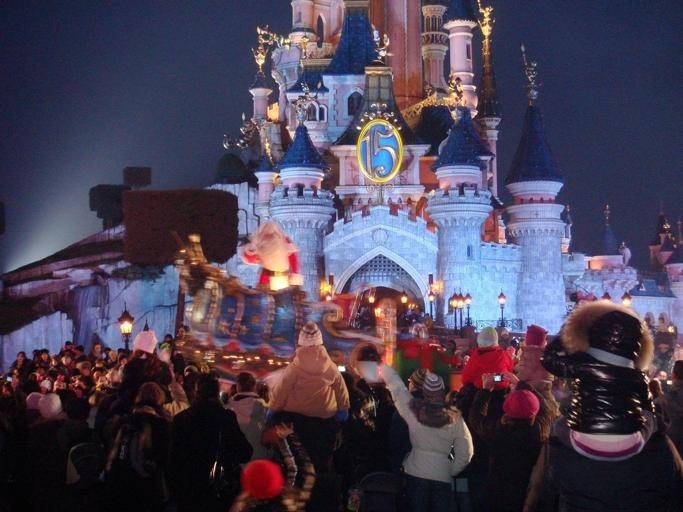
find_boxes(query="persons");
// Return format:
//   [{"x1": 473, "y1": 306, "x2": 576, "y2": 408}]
[
  {"x1": 241, "y1": 220, "x2": 306, "y2": 294},
  {"x1": 0, "y1": 301, "x2": 682, "y2": 512}
]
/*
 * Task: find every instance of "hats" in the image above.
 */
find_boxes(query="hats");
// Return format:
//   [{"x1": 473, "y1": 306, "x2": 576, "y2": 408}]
[
  {"x1": 299, "y1": 322, "x2": 323, "y2": 347},
  {"x1": 422, "y1": 374, "x2": 447, "y2": 399},
  {"x1": 503, "y1": 390, "x2": 539, "y2": 418},
  {"x1": 409, "y1": 369, "x2": 426, "y2": 388},
  {"x1": 26, "y1": 393, "x2": 43, "y2": 409},
  {"x1": 526, "y1": 325, "x2": 547, "y2": 346},
  {"x1": 132, "y1": 332, "x2": 157, "y2": 354},
  {"x1": 241, "y1": 459, "x2": 285, "y2": 500},
  {"x1": 476, "y1": 326, "x2": 498, "y2": 347},
  {"x1": 38, "y1": 394, "x2": 64, "y2": 419},
  {"x1": 40, "y1": 379, "x2": 52, "y2": 391}
]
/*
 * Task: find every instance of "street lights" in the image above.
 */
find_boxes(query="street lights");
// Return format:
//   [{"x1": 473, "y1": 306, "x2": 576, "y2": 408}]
[
  {"x1": 619, "y1": 290, "x2": 633, "y2": 307},
  {"x1": 450, "y1": 290, "x2": 459, "y2": 331},
  {"x1": 427, "y1": 289, "x2": 436, "y2": 319},
  {"x1": 456, "y1": 287, "x2": 463, "y2": 329},
  {"x1": 116, "y1": 297, "x2": 134, "y2": 349},
  {"x1": 398, "y1": 287, "x2": 409, "y2": 314},
  {"x1": 464, "y1": 292, "x2": 473, "y2": 326},
  {"x1": 497, "y1": 288, "x2": 506, "y2": 326},
  {"x1": 600, "y1": 289, "x2": 611, "y2": 302}
]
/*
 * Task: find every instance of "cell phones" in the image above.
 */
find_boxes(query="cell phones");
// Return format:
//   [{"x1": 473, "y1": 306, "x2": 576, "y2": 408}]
[
  {"x1": 337, "y1": 365, "x2": 346, "y2": 372},
  {"x1": 666, "y1": 380, "x2": 672, "y2": 386},
  {"x1": 493, "y1": 373, "x2": 503, "y2": 382}
]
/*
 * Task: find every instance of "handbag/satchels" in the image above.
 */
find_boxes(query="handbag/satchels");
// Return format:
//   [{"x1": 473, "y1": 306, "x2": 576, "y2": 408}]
[{"x1": 208, "y1": 452, "x2": 240, "y2": 503}]
